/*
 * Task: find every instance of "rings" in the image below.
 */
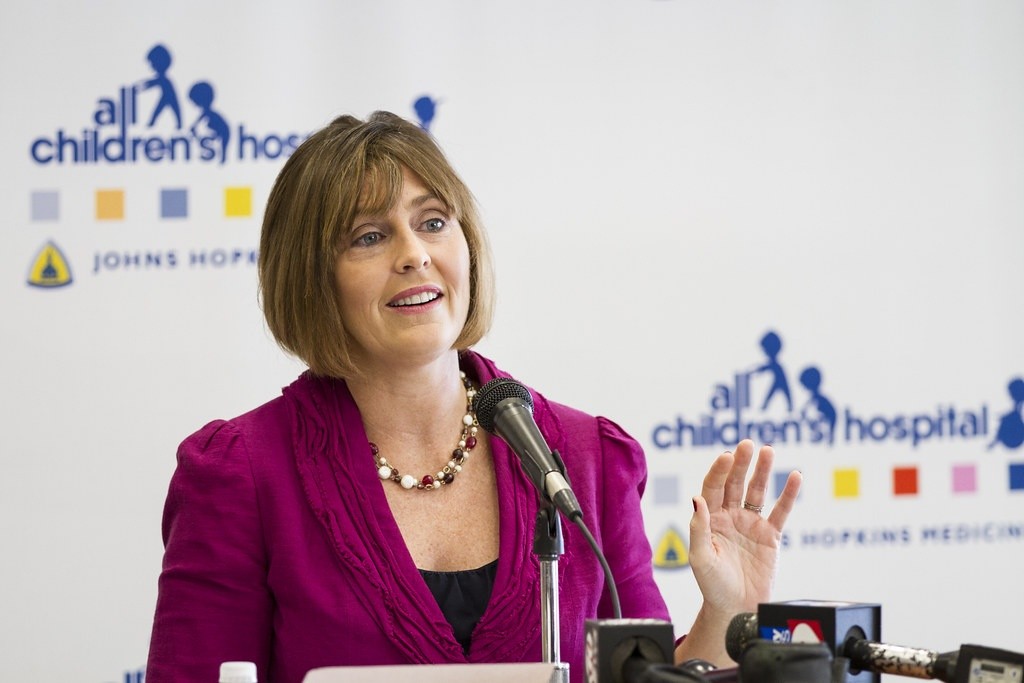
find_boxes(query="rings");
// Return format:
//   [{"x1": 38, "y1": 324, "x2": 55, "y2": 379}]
[{"x1": 744, "y1": 502, "x2": 764, "y2": 511}]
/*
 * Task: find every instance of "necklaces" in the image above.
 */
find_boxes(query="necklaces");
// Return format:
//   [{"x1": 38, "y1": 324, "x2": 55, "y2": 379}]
[{"x1": 368, "y1": 370, "x2": 480, "y2": 492}]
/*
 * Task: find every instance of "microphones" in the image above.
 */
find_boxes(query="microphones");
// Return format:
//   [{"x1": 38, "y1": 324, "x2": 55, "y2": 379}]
[
  {"x1": 583, "y1": 598, "x2": 1024, "y2": 683},
  {"x1": 470, "y1": 377, "x2": 582, "y2": 523}
]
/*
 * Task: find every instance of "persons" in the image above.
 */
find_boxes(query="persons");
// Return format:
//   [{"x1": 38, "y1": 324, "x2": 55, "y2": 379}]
[{"x1": 146, "y1": 110, "x2": 802, "y2": 683}]
